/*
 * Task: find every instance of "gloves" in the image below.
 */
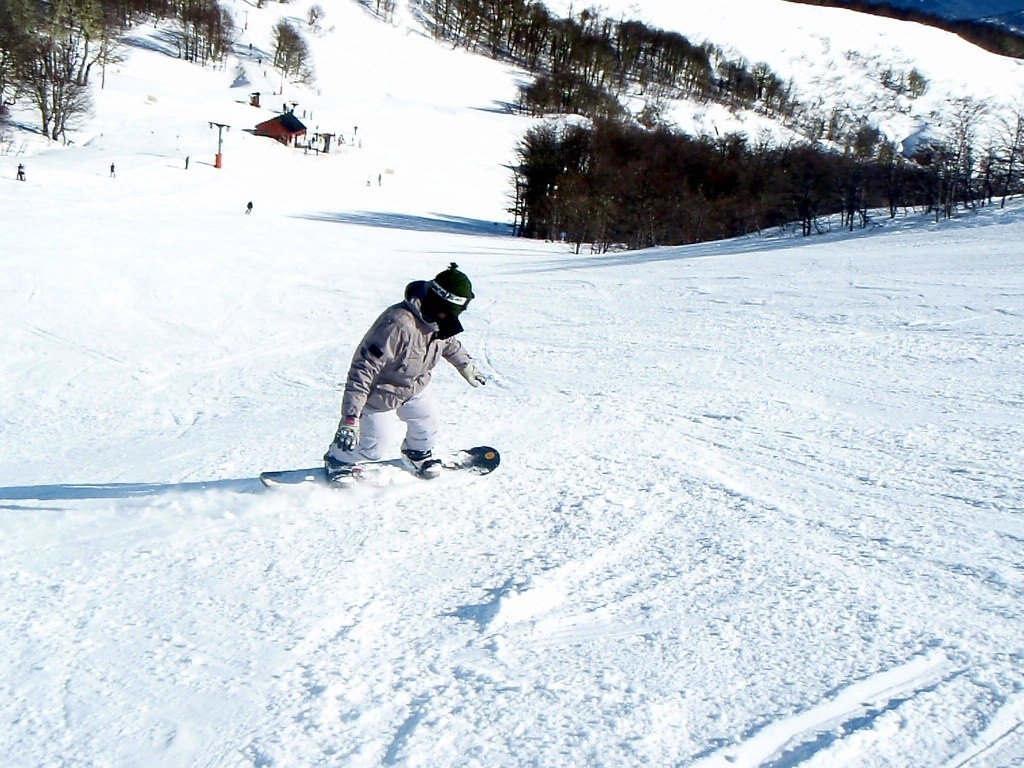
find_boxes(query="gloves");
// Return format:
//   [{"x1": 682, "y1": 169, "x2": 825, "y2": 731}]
[
  {"x1": 458, "y1": 361, "x2": 486, "y2": 387},
  {"x1": 334, "y1": 415, "x2": 360, "y2": 451}
]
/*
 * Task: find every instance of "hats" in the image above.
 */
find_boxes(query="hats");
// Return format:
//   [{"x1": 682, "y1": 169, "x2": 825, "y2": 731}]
[{"x1": 432, "y1": 262, "x2": 474, "y2": 311}]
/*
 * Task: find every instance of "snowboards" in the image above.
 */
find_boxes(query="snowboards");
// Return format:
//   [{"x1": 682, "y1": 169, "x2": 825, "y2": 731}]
[{"x1": 258, "y1": 444, "x2": 501, "y2": 491}]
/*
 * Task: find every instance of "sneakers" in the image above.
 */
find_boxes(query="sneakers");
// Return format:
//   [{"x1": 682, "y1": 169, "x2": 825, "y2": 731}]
[
  {"x1": 324, "y1": 452, "x2": 355, "y2": 489},
  {"x1": 401, "y1": 440, "x2": 441, "y2": 479}
]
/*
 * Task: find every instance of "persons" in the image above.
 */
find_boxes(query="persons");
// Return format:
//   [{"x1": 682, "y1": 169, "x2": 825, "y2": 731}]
[
  {"x1": 18, "y1": 163, "x2": 24, "y2": 180},
  {"x1": 185, "y1": 156, "x2": 190, "y2": 169},
  {"x1": 246, "y1": 201, "x2": 253, "y2": 213},
  {"x1": 111, "y1": 163, "x2": 115, "y2": 177},
  {"x1": 323, "y1": 268, "x2": 488, "y2": 489}
]
